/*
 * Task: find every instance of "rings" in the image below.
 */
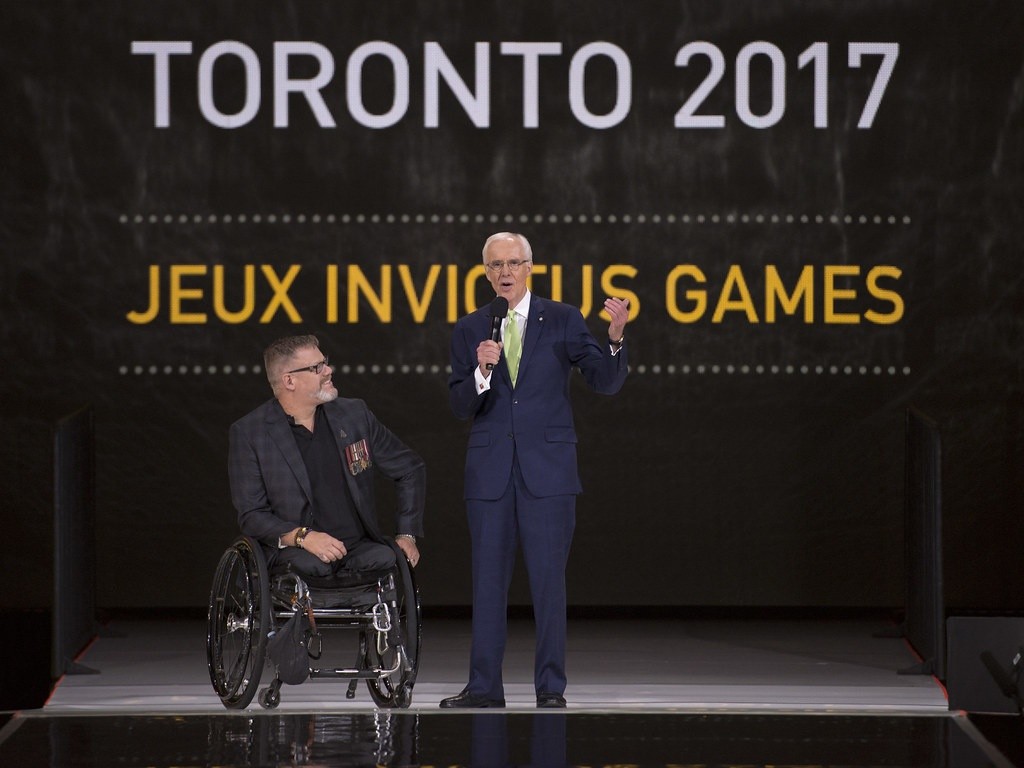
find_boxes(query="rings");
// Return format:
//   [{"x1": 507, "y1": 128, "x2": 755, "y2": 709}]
[{"x1": 409, "y1": 558, "x2": 416, "y2": 563}]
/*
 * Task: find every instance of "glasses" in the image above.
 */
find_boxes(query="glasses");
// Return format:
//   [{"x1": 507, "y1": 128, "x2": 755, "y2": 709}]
[
  {"x1": 486, "y1": 258, "x2": 530, "y2": 272},
  {"x1": 286, "y1": 356, "x2": 329, "y2": 375}
]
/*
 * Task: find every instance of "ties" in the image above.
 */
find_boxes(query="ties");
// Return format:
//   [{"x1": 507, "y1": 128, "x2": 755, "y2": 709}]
[{"x1": 504, "y1": 310, "x2": 522, "y2": 382}]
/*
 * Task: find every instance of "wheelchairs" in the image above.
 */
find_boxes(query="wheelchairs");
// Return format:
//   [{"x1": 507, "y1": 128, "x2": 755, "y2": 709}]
[{"x1": 205, "y1": 532, "x2": 423, "y2": 709}]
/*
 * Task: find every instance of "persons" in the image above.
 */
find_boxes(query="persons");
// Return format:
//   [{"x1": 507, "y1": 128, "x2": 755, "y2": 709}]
[
  {"x1": 439, "y1": 232, "x2": 629, "y2": 709},
  {"x1": 228, "y1": 334, "x2": 425, "y2": 588}
]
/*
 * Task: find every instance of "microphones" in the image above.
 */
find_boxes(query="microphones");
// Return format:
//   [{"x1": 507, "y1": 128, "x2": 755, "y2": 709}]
[{"x1": 486, "y1": 295, "x2": 509, "y2": 370}]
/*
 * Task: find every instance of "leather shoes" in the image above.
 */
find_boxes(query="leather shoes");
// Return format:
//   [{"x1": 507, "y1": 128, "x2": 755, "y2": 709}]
[
  {"x1": 537, "y1": 693, "x2": 566, "y2": 707},
  {"x1": 440, "y1": 691, "x2": 505, "y2": 708}
]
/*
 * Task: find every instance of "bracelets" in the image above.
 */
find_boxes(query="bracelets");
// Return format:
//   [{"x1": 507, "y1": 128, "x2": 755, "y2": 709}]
[
  {"x1": 395, "y1": 534, "x2": 417, "y2": 543},
  {"x1": 608, "y1": 330, "x2": 625, "y2": 345},
  {"x1": 293, "y1": 526, "x2": 313, "y2": 548}
]
